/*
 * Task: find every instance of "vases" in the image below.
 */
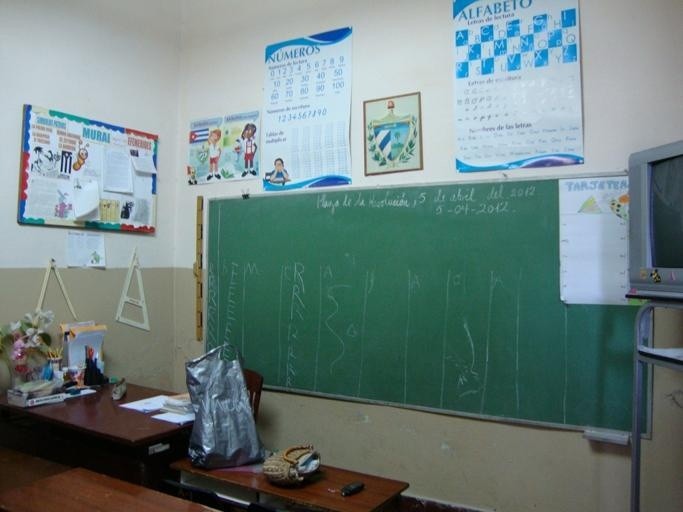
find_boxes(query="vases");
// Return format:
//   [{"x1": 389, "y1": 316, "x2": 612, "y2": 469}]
[{"x1": 9, "y1": 359, "x2": 27, "y2": 390}]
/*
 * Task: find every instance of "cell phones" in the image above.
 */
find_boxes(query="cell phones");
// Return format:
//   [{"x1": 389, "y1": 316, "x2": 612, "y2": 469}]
[{"x1": 340, "y1": 480, "x2": 365, "y2": 496}]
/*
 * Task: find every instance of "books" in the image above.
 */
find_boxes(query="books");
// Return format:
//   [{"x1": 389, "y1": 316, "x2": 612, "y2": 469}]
[
  {"x1": 6, "y1": 393, "x2": 65, "y2": 408},
  {"x1": 160, "y1": 392, "x2": 194, "y2": 415},
  {"x1": 56, "y1": 319, "x2": 110, "y2": 374}
]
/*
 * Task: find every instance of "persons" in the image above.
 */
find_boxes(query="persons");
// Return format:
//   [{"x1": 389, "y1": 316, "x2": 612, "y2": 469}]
[
  {"x1": 239, "y1": 122, "x2": 258, "y2": 176},
  {"x1": 204, "y1": 128, "x2": 223, "y2": 180},
  {"x1": 269, "y1": 158, "x2": 289, "y2": 183}
]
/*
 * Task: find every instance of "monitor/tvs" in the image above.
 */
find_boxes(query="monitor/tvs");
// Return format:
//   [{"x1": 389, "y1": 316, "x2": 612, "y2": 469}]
[{"x1": 626, "y1": 140, "x2": 683, "y2": 301}]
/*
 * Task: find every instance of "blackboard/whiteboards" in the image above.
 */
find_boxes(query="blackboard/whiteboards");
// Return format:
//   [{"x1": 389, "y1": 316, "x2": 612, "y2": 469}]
[{"x1": 208, "y1": 174, "x2": 651, "y2": 444}]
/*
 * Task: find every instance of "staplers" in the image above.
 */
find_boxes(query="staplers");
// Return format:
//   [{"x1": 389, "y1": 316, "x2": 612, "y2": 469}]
[{"x1": 108, "y1": 377, "x2": 126, "y2": 400}]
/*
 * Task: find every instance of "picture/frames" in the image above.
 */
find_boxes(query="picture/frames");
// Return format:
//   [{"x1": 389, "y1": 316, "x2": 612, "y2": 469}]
[{"x1": 364, "y1": 92, "x2": 422, "y2": 176}]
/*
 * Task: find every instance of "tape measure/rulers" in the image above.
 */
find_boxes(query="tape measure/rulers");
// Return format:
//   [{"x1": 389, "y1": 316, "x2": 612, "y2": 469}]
[{"x1": 115, "y1": 247, "x2": 151, "y2": 332}]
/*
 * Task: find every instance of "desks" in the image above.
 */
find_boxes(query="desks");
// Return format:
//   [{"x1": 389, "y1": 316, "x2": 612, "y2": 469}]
[
  {"x1": 1, "y1": 380, "x2": 196, "y2": 485},
  {"x1": 1, "y1": 463, "x2": 218, "y2": 511},
  {"x1": 169, "y1": 448, "x2": 409, "y2": 511}
]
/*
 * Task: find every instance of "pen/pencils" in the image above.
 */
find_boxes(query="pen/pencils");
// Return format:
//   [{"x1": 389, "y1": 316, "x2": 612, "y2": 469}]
[{"x1": 48, "y1": 347, "x2": 63, "y2": 358}]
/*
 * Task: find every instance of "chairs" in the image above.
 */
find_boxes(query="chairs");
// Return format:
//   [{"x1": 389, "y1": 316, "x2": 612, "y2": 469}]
[{"x1": 239, "y1": 369, "x2": 262, "y2": 427}]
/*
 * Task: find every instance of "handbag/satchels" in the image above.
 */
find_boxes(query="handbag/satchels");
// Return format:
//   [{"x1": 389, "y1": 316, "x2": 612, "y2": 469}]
[{"x1": 263, "y1": 446, "x2": 321, "y2": 485}]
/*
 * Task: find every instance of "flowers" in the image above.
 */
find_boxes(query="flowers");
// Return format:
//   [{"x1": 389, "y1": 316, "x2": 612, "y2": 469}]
[{"x1": 0, "y1": 308, "x2": 56, "y2": 371}]
